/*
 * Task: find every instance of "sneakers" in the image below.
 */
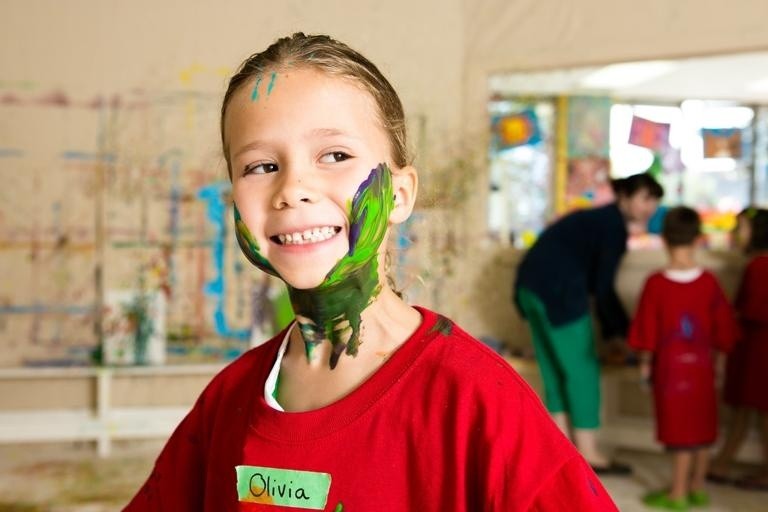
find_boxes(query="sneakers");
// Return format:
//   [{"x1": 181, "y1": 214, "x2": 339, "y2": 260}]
[
  {"x1": 643, "y1": 488, "x2": 709, "y2": 511},
  {"x1": 593, "y1": 462, "x2": 630, "y2": 474}
]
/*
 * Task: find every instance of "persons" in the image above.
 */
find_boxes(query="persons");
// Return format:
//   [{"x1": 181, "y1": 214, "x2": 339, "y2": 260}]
[
  {"x1": 703, "y1": 205, "x2": 767, "y2": 494},
  {"x1": 510, "y1": 171, "x2": 664, "y2": 477},
  {"x1": 122, "y1": 30, "x2": 623, "y2": 512},
  {"x1": 628, "y1": 204, "x2": 749, "y2": 512}
]
